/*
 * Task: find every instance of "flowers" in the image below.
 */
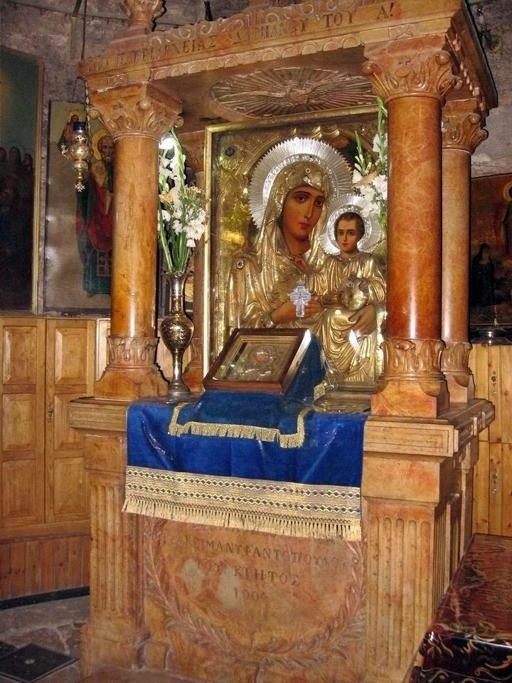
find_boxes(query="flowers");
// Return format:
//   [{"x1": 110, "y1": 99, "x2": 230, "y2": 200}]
[{"x1": 152, "y1": 125, "x2": 206, "y2": 273}]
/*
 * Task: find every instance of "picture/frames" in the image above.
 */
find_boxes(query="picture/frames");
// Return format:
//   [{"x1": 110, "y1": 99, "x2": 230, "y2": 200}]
[
  {"x1": 202, "y1": 326, "x2": 312, "y2": 395},
  {"x1": 0, "y1": 42, "x2": 44, "y2": 316}
]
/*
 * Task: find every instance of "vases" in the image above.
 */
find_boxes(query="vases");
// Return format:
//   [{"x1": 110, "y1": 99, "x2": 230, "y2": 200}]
[{"x1": 162, "y1": 274, "x2": 194, "y2": 398}]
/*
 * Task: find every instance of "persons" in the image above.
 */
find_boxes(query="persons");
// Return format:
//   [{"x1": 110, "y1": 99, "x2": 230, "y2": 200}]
[
  {"x1": 6, "y1": 144, "x2": 23, "y2": 205},
  {"x1": 0, "y1": 146, "x2": 13, "y2": 205},
  {"x1": 21, "y1": 151, "x2": 35, "y2": 203},
  {"x1": 72, "y1": 127, "x2": 115, "y2": 296},
  {"x1": 300, "y1": 203, "x2": 387, "y2": 391},
  {"x1": 470, "y1": 238, "x2": 497, "y2": 316},
  {"x1": 223, "y1": 132, "x2": 354, "y2": 332}
]
